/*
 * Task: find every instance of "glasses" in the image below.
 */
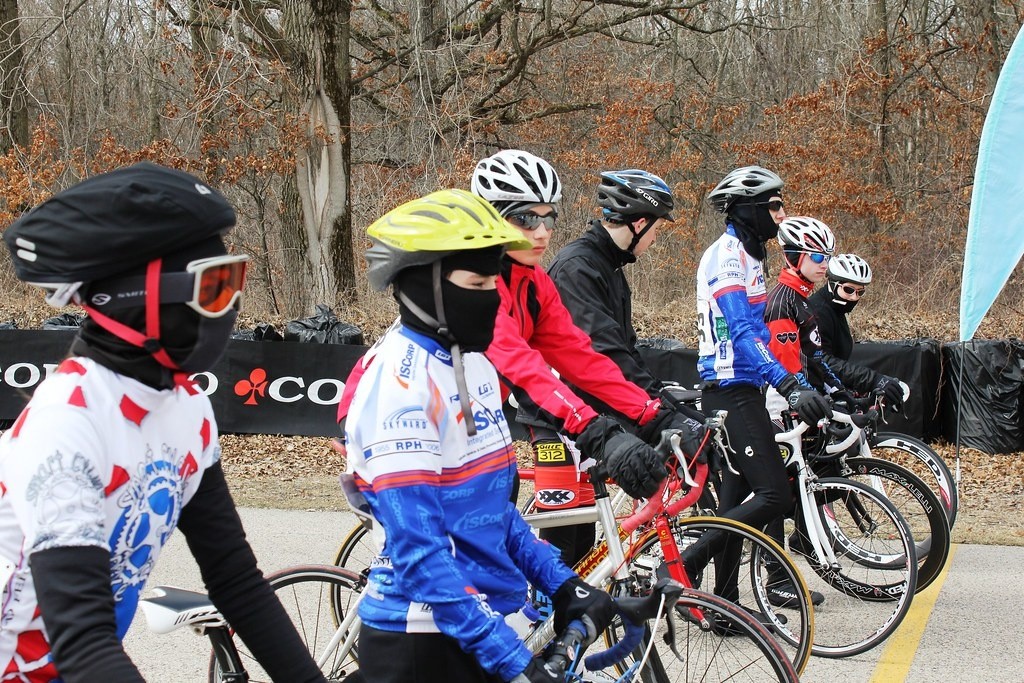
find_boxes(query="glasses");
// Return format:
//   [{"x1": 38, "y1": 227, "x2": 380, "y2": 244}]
[
  {"x1": 783, "y1": 250, "x2": 831, "y2": 263},
  {"x1": 736, "y1": 201, "x2": 783, "y2": 211},
  {"x1": 506, "y1": 212, "x2": 557, "y2": 231},
  {"x1": 187, "y1": 253, "x2": 249, "y2": 319},
  {"x1": 837, "y1": 284, "x2": 866, "y2": 297}
]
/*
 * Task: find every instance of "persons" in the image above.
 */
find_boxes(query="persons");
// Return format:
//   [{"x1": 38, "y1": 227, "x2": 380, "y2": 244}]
[
  {"x1": 514, "y1": 169, "x2": 705, "y2": 659},
  {"x1": 657, "y1": 165, "x2": 904, "y2": 637},
  {"x1": 471, "y1": 147, "x2": 720, "y2": 508},
  {"x1": 0, "y1": 160, "x2": 327, "y2": 683},
  {"x1": 336, "y1": 187, "x2": 619, "y2": 683}
]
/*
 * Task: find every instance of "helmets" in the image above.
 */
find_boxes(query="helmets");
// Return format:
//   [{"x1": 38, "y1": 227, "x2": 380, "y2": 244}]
[
  {"x1": 471, "y1": 150, "x2": 562, "y2": 203},
  {"x1": 364, "y1": 190, "x2": 531, "y2": 293},
  {"x1": 777, "y1": 216, "x2": 836, "y2": 254},
  {"x1": 598, "y1": 169, "x2": 675, "y2": 223},
  {"x1": 707, "y1": 167, "x2": 784, "y2": 214},
  {"x1": 2, "y1": 162, "x2": 237, "y2": 308},
  {"x1": 825, "y1": 254, "x2": 872, "y2": 285}
]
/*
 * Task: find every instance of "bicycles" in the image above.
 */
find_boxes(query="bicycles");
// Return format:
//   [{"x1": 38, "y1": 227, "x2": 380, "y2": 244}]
[
  {"x1": 207, "y1": 373, "x2": 960, "y2": 682},
  {"x1": 138, "y1": 574, "x2": 688, "y2": 683}
]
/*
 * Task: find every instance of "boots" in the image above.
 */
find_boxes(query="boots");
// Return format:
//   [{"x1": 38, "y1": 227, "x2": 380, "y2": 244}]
[
  {"x1": 713, "y1": 587, "x2": 787, "y2": 636},
  {"x1": 656, "y1": 546, "x2": 707, "y2": 623},
  {"x1": 788, "y1": 521, "x2": 838, "y2": 556},
  {"x1": 765, "y1": 562, "x2": 824, "y2": 608}
]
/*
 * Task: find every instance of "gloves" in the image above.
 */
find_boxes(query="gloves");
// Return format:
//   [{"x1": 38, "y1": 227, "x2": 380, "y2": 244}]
[
  {"x1": 576, "y1": 416, "x2": 667, "y2": 500},
  {"x1": 654, "y1": 404, "x2": 721, "y2": 484},
  {"x1": 509, "y1": 661, "x2": 566, "y2": 683},
  {"x1": 552, "y1": 575, "x2": 619, "y2": 649},
  {"x1": 778, "y1": 373, "x2": 833, "y2": 427},
  {"x1": 829, "y1": 385, "x2": 861, "y2": 416},
  {"x1": 873, "y1": 372, "x2": 904, "y2": 404}
]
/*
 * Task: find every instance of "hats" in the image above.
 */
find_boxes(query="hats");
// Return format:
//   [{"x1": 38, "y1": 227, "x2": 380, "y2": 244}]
[{"x1": 493, "y1": 201, "x2": 558, "y2": 220}]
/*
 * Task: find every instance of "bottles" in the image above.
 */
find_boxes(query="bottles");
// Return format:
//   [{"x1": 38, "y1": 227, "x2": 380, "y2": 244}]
[{"x1": 504, "y1": 602, "x2": 541, "y2": 640}]
